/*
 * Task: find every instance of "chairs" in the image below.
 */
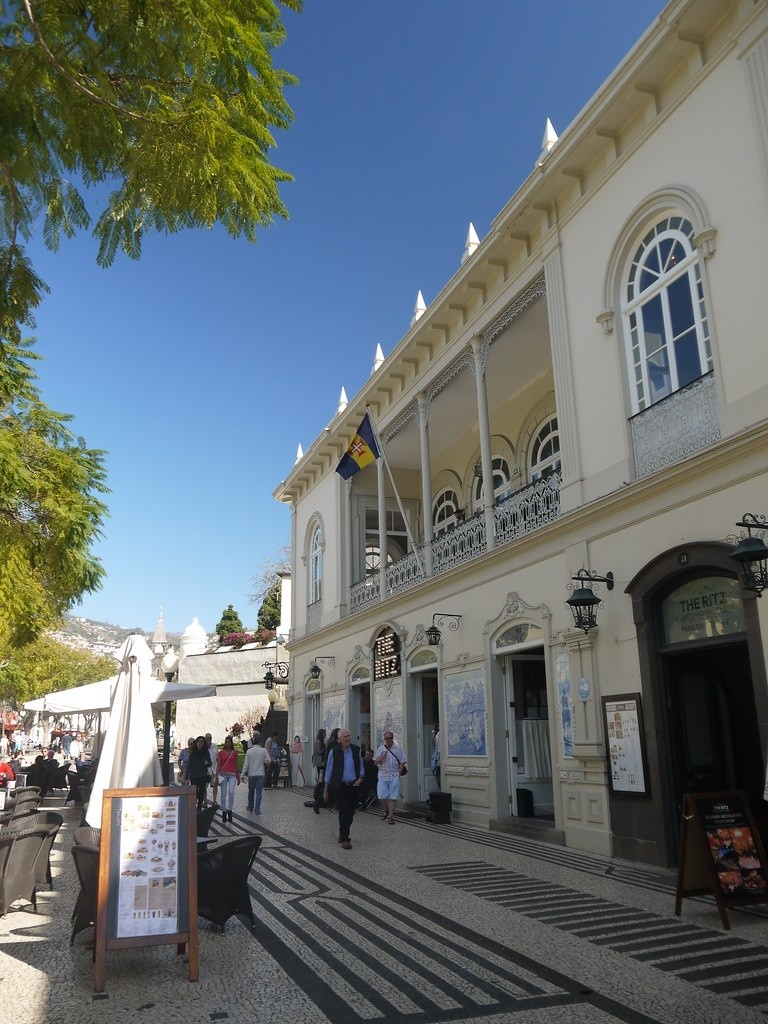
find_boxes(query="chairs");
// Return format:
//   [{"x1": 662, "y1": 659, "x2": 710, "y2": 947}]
[
  {"x1": 195, "y1": 804, "x2": 219, "y2": 836},
  {"x1": 197, "y1": 836, "x2": 261, "y2": 936},
  {"x1": 0, "y1": 763, "x2": 100, "y2": 946}
]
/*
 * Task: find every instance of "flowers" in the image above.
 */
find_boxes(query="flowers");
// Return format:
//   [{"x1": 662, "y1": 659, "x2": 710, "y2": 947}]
[{"x1": 223, "y1": 629, "x2": 272, "y2": 646}]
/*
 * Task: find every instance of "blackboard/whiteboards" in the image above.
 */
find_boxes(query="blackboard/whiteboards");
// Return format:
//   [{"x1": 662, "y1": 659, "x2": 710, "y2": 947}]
[{"x1": 95, "y1": 786, "x2": 198, "y2": 949}]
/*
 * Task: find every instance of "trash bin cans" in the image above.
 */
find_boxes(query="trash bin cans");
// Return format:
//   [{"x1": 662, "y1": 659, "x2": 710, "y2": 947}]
[{"x1": 516, "y1": 789, "x2": 534, "y2": 817}]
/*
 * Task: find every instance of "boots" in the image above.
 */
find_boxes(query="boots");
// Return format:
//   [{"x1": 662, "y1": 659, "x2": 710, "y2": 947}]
[
  {"x1": 223, "y1": 811, "x2": 227, "y2": 823},
  {"x1": 228, "y1": 810, "x2": 232, "y2": 822}
]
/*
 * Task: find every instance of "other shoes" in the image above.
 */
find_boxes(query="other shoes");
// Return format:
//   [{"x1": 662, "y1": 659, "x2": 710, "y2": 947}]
[
  {"x1": 340, "y1": 841, "x2": 352, "y2": 849},
  {"x1": 203, "y1": 802, "x2": 208, "y2": 808},
  {"x1": 212, "y1": 802, "x2": 217, "y2": 807}
]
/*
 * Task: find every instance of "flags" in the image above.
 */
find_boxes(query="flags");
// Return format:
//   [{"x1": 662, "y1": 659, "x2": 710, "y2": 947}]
[{"x1": 335, "y1": 413, "x2": 380, "y2": 481}]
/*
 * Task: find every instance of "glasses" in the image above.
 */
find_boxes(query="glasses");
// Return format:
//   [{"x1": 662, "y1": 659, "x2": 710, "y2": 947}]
[{"x1": 384, "y1": 737, "x2": 393, "y2": 740}]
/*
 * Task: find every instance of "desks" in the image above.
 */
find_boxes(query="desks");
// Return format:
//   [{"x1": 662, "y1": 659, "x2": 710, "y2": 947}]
[{"x1": 197, "y1": 835, "x2": 217, "y2": 850}]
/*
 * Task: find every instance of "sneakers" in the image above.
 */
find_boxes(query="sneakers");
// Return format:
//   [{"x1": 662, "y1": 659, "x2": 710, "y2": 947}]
[
  {"x1": 246, "y1": 806, "x2": 252, "y2": 812},
  {"x1": 255, "y1": 811, "x2": 261, "y2": 815}
]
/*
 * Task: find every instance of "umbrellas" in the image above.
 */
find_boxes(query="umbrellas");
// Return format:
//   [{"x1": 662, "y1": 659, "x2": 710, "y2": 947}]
[
  {"x1": 23, "y1": 675, "x2": 215, "y2": 714},
  {"x1": 83, "y1": 632, "x2": 165, "y2": 833}
]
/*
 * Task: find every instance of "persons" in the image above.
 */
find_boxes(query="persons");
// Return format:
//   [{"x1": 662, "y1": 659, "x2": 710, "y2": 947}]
[
  {"x1": 323, "y1": 729, "x2": 366, "y2": 849},
  {"x1": 247, "y1": 731, "x2": 260, "y2": 748},
  {"x1": 289, "y1": 735, "x2": 305, "y2": 786},
  {"x1": 372, "y1": 732, "x2": 407, "y2": 824},
  {"x1": 356, "y1": 749, "x2": 379, "y2": 811},
  {"x1": 178, "y1": 733, "x2": 218, "y2": 811},
  {"x1": 216, "y1": 737, "x2": 240, "y2": 822},
  {"x1": 430, "y1": 730, "x2": 441, "y2": 789},
  {"x1": 265, "y1": 731, "x2": 280, "y2": 790},
  {"x1": 239, "y1": 735, "x2": 271, "y2": 815},
  {"x1": 0, "y1": 730, "x2": 94, "y2": 787},
  {"x1": 313, "y1": 728, "x2": 340, "y2": 782}
]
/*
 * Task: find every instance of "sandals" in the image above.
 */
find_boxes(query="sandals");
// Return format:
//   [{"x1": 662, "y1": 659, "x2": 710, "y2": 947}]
[
  {"x1": 381, "y1": 811, "x2": 388, "y2": 820},
  {"x1": 387, "y1": 818, "x2": 394, "y2": 825}
]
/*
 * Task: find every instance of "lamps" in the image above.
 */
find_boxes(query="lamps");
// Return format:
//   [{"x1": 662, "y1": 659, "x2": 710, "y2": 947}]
[
  {"x1": 261, "y1": 661, "x2": 291, "y2": 690},
  {"x1": 727, "y1": 513, "x2": 768, "y2": 600},
  {"x1": 311, "y1": 657, "x2": 334, "y2": 679},
  {"x1": 566, "y1": 568, "x2": 614, "y2": 634},
  {"x1": 454, "y1": 508, "x2": 466, "y2": 523},
  {"x1": 426, "y1": 612, "x2": 462, "y2": 646}
]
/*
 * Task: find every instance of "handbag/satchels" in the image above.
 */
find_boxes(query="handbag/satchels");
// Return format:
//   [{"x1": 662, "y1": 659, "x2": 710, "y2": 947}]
[
  {"x1": 216, "y1": 773, "x2": 225, "y2": 784},
  {"x1": 399, "y1": 764, "x2": 407, "y2": 775}
]
